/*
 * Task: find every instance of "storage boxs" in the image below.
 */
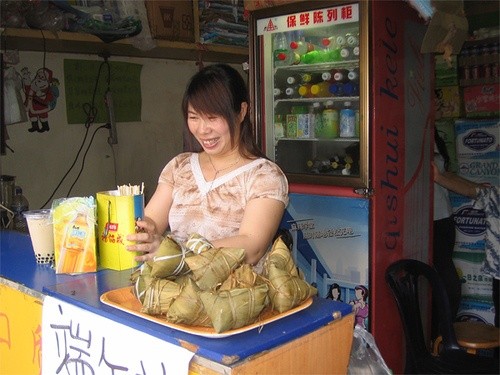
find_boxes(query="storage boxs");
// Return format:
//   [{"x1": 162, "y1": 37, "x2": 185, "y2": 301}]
[{"x1": 432, "y1": 39, "x2": 500, "y2": 360}]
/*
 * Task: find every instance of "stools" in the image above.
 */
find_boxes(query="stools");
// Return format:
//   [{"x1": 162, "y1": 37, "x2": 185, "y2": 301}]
[{"x1": 432, "y1": 321, "x2": 500, "y2": 357}]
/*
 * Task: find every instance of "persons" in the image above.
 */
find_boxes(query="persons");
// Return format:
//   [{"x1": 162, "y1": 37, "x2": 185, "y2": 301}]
[
  {"x1": 432, "y1": 88, "x2": 494, "y2": 342},
  {"x1": 125, "y1": 64, "x2": 289, "y2": 266}
]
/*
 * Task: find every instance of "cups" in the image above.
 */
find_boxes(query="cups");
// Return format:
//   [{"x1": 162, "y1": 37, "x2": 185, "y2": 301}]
[{"x1": 21, "y1": 208, "x2": 55, "y2": 265}]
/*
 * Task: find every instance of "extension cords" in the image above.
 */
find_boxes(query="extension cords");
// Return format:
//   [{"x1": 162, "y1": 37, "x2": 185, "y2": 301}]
[{"x1": 104, "y1": 92, "x2": 118, "y2": 144}]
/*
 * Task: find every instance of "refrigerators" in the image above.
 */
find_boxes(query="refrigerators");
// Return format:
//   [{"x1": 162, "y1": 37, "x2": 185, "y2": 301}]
[{"x1": 247, "y1": 0, "x2": 434, "y2": 375}]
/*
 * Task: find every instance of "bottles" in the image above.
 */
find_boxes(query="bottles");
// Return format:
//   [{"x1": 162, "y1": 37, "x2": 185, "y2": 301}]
[{"x1": 273, "y1": 29, "x2": 361, "y2": 176}]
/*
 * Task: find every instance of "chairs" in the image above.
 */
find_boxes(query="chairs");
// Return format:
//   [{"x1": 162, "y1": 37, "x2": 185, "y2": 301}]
[{"x1": 385, "y1": 258, "x2": 500, "y2": 375}]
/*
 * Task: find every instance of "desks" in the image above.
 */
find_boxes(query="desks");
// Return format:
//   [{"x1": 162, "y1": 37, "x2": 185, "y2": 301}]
[{"x1": 0, "y1": 228, "x2": 356, "y2": 375}]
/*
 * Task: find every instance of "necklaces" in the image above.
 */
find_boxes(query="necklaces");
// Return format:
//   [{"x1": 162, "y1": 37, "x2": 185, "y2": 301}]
[{"x1": 207, "y1": 154, "x2": 249, "y2": 192}]
